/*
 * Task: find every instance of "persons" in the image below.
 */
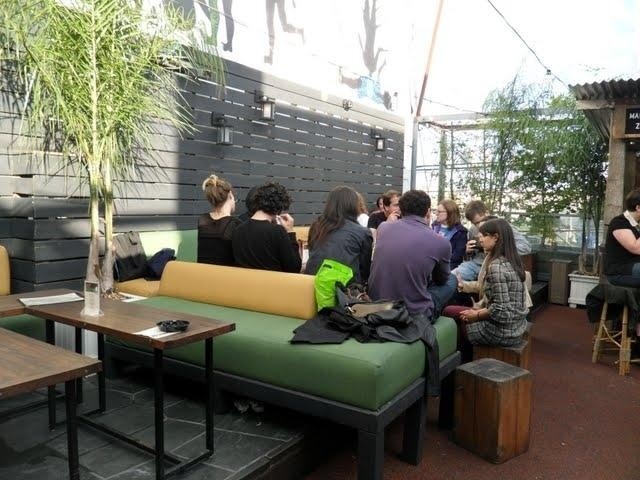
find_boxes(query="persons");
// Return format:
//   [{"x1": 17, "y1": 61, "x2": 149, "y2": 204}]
[
  {"x1": 305, "y1": 184, "x2": 532, "y2": 324},
  {"x1": 444, "y1": 217, "x2": 530, "y2": 354},
  {"x1": 605, "y1": 189, "x2": 639, "y2": 365},
  {"x1": 160, "y1": 0, "x2": 416, "y2": 115},
  {"x1": 195, "y1": 173, "x2": 304, "y2": 274}
]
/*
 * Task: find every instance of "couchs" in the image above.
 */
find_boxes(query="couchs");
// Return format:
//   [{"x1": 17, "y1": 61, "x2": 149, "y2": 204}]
[
  {"x1": 97, "y1": 261, "x2": 464, "y2": 480},
  {"x1": 113, "y1": 227, "x2": 315, "y2": 296}
]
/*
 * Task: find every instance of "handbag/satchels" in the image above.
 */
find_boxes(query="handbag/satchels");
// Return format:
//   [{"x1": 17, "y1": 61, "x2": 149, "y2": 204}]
[
  {"x1": 144, "y1": 248, "x2": 176, "y2": 280},
  {"x1": 111, "y1": 231, "x2": 147, "y2": 282},
  {"x1": 335, "y1": 281, "x2": 412, "y2": 327},
  {"x1": 314, "y1": 260, "x2": 352, "y2": 312}
]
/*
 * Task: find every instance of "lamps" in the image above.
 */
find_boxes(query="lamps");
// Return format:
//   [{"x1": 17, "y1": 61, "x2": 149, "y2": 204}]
[
  {"x1": 254, "y1": 89, "x2": 275, "y2": 121},
  {"x1": 370, "y1": 129, "x2": 386, "y2": 151},
  {"x1": 211, "y1": 112, "x2": 234, "y2": 146}
]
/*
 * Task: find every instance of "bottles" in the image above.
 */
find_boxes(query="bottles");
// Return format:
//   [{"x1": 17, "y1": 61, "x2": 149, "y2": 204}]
[{"x1": 296, "y1": 234, "x2": 303, "y2": 266}]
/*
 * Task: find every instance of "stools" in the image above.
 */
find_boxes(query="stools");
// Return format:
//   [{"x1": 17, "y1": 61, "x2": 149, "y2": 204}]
[
  {"x1": 454, "y1": 355, "x2": 534, "y2": 464},
  {"x1": 590, "y1": 284, "x2": 640, "y2": 376},
  {"x1": 472, "y1": 340, "x2": 532, "y2": 369}
]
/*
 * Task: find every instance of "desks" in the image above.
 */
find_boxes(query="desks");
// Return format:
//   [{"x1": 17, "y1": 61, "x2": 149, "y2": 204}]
[
  {"x1": 548, "y1": 258, "x2": 573, "y2": 306},
  {"x1": 1, "y1": 328, "x2": 104, "y2": 480},
  {"x1": 1, "y1": 284, "x2": 237, "y2": 480}
]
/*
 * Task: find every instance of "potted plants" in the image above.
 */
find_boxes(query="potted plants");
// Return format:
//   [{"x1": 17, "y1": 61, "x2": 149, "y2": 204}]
[{"x1": 546, "y1": 124, "x2": 608, "y2": 309}]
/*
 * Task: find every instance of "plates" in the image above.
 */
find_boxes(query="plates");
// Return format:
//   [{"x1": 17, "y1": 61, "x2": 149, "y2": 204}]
[{"x1": 156, "y1": 318, "x2": 190, "y2": 332}]
